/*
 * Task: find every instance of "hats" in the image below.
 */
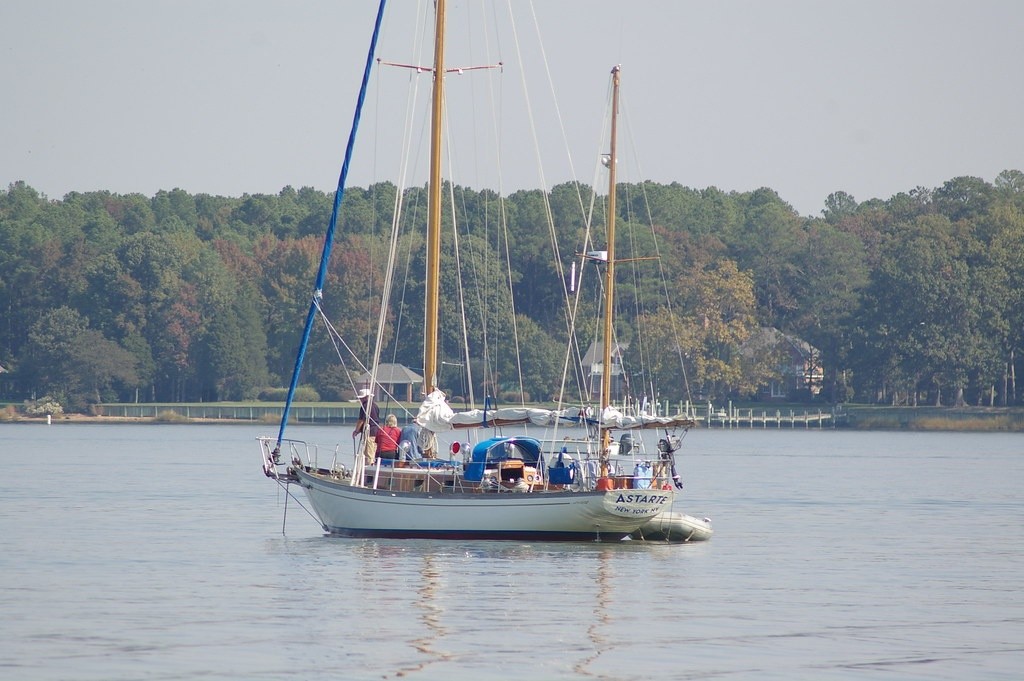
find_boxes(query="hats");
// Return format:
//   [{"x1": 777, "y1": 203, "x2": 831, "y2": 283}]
[{"x1": 356, "y1": 388, "x2": 375, "y2": 398}]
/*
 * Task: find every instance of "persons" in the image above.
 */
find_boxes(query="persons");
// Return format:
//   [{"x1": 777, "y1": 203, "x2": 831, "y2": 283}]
[
  {"x1": 352, "y1": 389, "x2": 379, "y2": 465},
  {"x1": 375, "y1": 414, "x2": 401, "y2": 459},
  {"x1": 400, "y1": 417, "x2": 424, "y2": 460}
]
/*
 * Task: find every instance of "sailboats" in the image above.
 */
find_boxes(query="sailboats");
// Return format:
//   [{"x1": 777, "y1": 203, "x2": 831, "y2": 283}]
[{"x1": 256, "y1": 1, "x2": 714, "y2": 549}]
